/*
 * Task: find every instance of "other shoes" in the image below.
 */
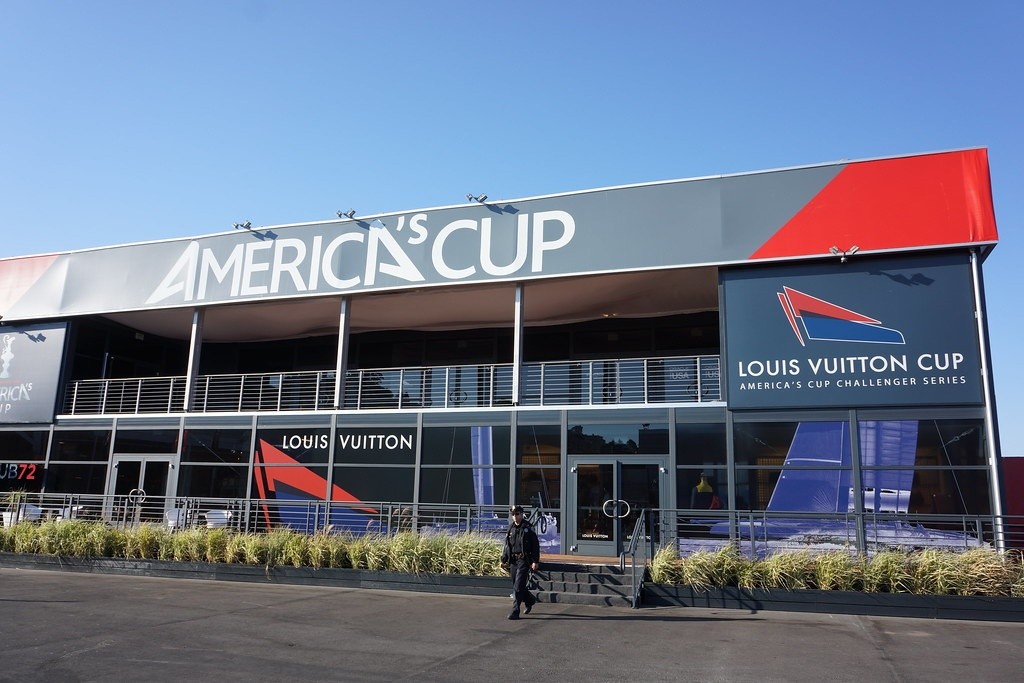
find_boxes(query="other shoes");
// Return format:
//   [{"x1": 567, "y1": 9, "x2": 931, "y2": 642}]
[
  {"x1": 523, "y1": 596, "x2": 535, "y2": 614},
  {"x1": 507, "y1": 611, "x2": 519, "y2": 619}
]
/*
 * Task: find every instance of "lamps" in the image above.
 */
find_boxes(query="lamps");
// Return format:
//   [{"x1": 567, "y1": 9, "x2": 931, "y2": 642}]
[
  {"x1": 466, "y1": 193, "x2": 488, "y2": 203},
  {"x1": 336, "y1": 209, "x2": 355, "y2": 218},
  {"x1": 231, "y1": 221, "x2": 252, "y2": 230},
  {"x1": 828, "y1": 245, "x2": 860, "y2": 262}
]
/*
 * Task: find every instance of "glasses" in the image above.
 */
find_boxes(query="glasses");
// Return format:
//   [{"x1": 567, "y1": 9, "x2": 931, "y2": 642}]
[{"x1": 511, "y1": 512, "x2": 523, "y2": 516}]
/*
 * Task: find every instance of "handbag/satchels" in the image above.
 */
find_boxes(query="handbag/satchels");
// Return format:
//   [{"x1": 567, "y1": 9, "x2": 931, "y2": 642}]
[{"x1": 501, "y1": 522, "x2": 514, "y2": 564}]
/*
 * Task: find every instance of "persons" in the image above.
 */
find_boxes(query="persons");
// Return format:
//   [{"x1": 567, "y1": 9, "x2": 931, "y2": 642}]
[{"x1": 500, "y1": 505, "x2": 540, "y2": 620}]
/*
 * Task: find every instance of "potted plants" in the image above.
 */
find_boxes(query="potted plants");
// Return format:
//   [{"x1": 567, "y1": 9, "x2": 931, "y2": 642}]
[{"x1": 3, "y1": 487, "x2": 28, "y2": 529}]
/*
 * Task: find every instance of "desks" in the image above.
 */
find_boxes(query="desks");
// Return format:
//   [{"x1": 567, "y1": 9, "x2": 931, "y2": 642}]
[{"x1": 38, "y1": 507, "x2": 63, "y2": 521}]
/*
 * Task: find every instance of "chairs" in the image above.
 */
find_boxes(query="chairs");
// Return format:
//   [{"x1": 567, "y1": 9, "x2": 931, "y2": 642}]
[
  {"x1": 166, "y1": 508, "x2": 190, "y2": 536},
  {"x1": 57, "y1": 505, "x2": 86, "y2": 523},
  {"x1": 16, "y1": 503, "x2": 42, "y2": 524},
  {"x1": 205, "y1": 509, "x2": 232, "y2": 529}
]
